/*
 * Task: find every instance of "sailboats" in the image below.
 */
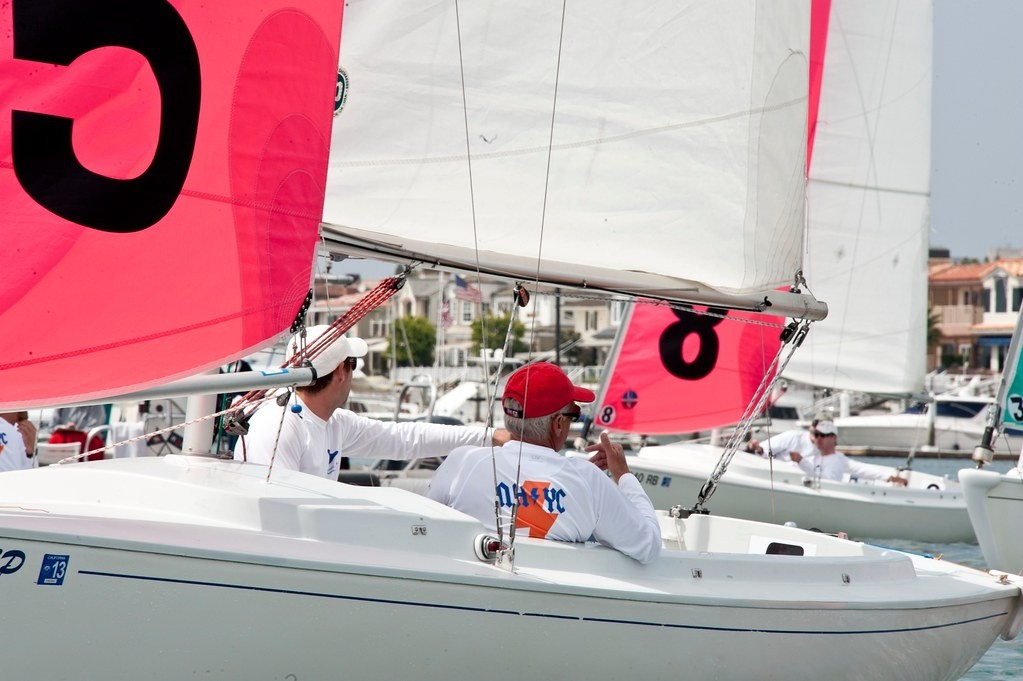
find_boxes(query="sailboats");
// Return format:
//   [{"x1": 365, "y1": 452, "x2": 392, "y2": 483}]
[{"x1": 2, "y1": 3, "x2": 1023, "y2": 681}]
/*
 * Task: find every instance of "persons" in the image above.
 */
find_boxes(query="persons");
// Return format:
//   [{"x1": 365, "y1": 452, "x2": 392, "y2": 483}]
[
  {"x1": 425, "y1": 362, "x2": 662, "y2": 572},
  {"x1": 48, "y1": 405, "x2": 106, "y2": 440},
  {"x1": 212, "y1": 325, "x2": 511, "y2": 482},
  {"x1": 0, "y1": 412, "x2": 38, "y2": 472},
  {"x1": 747, "y1": 412, "x2": 908, "y2": 486}
]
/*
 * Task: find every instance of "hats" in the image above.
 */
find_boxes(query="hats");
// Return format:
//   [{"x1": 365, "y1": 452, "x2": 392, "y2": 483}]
[
  {"x1": 502, "y1": 363, "x2": 595, "y2": 419},
  {"x1": 816, "y1": 420, "x2": 838, "y2": 435},
  {"x1": 285, "y1": 325, "x2": 368, "y2": 378}
]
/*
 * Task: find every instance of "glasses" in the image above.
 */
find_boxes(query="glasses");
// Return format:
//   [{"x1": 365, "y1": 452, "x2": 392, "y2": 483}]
[
  {"x1": 814, "y1": 431, "x2": 833, "y2": 439},
  {"x1": 550, "y1": 404, "x2": 581, "y2": 422},
  {"x1": 345, "y1": 357, "x2": 357, "y2": 371}
]
